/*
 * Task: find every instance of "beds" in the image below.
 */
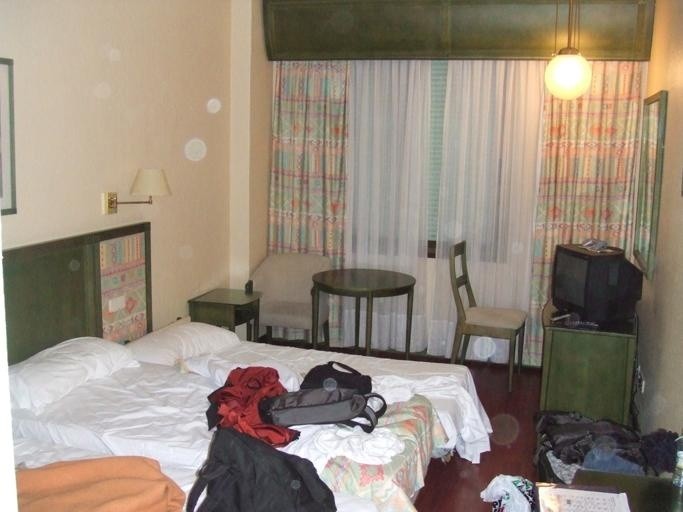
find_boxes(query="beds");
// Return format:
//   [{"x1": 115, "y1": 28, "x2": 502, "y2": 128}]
[
  {"x1": 12, "y1": 437, "x2": 419, "y2": 512},
  {"x1": 8, "y1": 336, "x2": 449, "y2": 505},
  {"x1": 122, "y1": 315, "x2": 492, "y2": 465}
]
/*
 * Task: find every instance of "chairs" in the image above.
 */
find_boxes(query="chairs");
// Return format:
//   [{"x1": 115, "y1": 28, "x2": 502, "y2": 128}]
[
  {"x1": 449, "y1": 240, "x2": 529, "y2": 395},
  {"x1": 250, "y1": 252, "x2": 331, "y2": 351}
]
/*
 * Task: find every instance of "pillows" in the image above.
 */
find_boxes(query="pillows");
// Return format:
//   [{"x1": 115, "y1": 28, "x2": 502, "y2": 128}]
[
  {"x1": 8, "y1": 335, "x2": 134, "y2": 412},
  {"x1": 123, "y1": 320, "x2": 238, "y2": 368}
]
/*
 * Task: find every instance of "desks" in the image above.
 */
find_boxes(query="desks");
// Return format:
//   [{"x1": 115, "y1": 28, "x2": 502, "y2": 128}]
[
  {"x1": 539, "y1": 300, "x2": 638, "y2": 428},
  {"x1": 552, "y1": 468, "x2": 683, "y2": 510}
]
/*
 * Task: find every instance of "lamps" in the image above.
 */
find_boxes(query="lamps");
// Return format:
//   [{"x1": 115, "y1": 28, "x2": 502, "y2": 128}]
[
  {"x1": 544, "y1": 1, "x2": 592, "y2": 100},
  {"x1": 100, "y1": 168, "x2": 171, "y2": 216}
]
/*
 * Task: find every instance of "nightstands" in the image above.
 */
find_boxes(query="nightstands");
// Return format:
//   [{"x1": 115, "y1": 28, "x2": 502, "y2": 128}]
[{"x1": 185, "y1": 286, "x2": 261, "y2": 342}]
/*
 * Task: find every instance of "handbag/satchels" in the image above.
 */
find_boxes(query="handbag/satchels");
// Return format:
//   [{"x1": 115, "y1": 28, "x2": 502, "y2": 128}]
[{"x1": 300, "y1": 360, "x2": 372, "y2": 395}]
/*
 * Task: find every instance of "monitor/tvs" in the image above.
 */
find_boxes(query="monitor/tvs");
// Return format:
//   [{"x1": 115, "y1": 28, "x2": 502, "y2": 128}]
[{"x1": 549, "y1": 241, "x2": 643, "y2": 326}]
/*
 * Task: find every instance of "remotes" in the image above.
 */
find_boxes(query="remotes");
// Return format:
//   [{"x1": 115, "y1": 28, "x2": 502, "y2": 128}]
[{"x1": 563, "y1": 319, "x2": 600, "y2": 331}]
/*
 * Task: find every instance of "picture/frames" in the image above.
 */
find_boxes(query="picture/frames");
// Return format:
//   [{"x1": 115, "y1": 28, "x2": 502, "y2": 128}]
[
  {"x1": 1, "y1": 58, "x2": 17, "y2": 215},
  {"x1": 632, "y1": 90, "x2": 667, "y2": 282}
]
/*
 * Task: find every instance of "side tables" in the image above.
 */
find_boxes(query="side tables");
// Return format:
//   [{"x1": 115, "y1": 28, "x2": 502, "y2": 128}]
[{"x1": 312, "y1": 270, "x2": 416, "y2": 360}]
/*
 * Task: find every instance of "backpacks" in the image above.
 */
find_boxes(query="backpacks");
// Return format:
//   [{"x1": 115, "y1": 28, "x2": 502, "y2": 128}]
[
  {"x1": 271, "y1": 386, "x2": 387, "y2": 433},
  {"x1": 186, "y1": 426, "x2": 336, "y2": 512}
]
